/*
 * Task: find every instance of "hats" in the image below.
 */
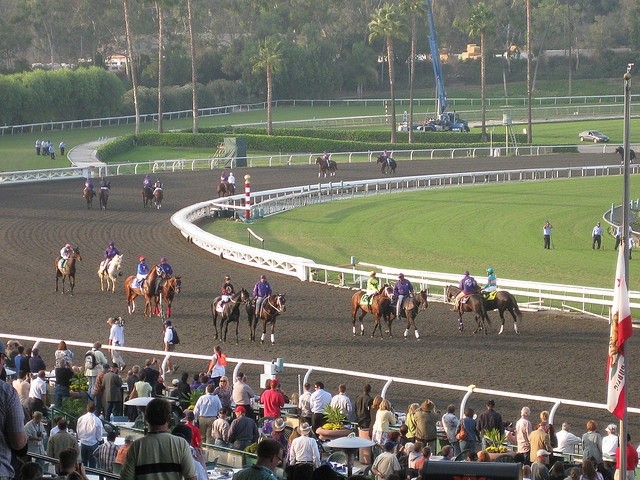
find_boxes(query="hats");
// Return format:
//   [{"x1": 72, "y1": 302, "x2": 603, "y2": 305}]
[
  {"x1": 536, "y1": 449, "x2": 551, "y2": 457},
  {"x1": 270, "y1": 379, "x2": 279, "y2": 386},
  {"x1": 31, "y1": 411, "x2": 46, "y2": 419},
  {"x1": 384, "y1": 441, "x2": 396, "y2": 450},
  {"x1": 161, "y1": 258, "x2": 167, "y2": 262},
  {"x1": 0, "y1": 342, "x2": 11, "y2": 362},
  {"x1": 260, "y1": 275, "x2": 266, "y2": 279},
  {"x1": 59, "y1": 448, "x2": 78, "y2": 466},
  {"x1": 370, "y1": 271, "x2": 376, "y2": 277},
  {"x1": 271, "y1": 418, "x2": 286, "y2": 432},
  {"x1": 232, "y1": 406, "x2": 246, "y2": 413},
  {"x1": 106, "y1": 317, "x2": 115, "y2": 323},
  {"x1": 421, "y1": 399, "x2": 434, "y2": 411},
  {"x1": 296, "y1": 422, "x2": 312, "y2": 436},
  {"x1": 139, "y1": 256, "x2": 146, "y2": 261},
  {"x1": 604, "y1": 424, "x2": 618, "y2": 435},
  {"x1": 398, "y1": 273, "x2": 404, "y2": 278}
]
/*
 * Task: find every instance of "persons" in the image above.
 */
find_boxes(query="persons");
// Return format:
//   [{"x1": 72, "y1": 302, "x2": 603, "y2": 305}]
[
  {"x1": 136, "y1": 255, "x2": 151, "y2": 292},
  {"x1": 372, "y1": 441, "x2": 401, "y2": 480},
  {"x1": 199, "y1": 372, "x2": 215, "y2": 386},
  {"x1": 154, "y1": 255, "x2": 173, "y2": 295},
  {"x1": 60, "y1": 244, "x2": 74, "y2": 268},
  {"x1": 153, "y1": 376, "x2": 170, "y2": 397},
  {"x1": 330, "y1": 384, "x2": 353, "y2": 425},
  {"x1": 501, "y1": 417, "x2": 518, "y2": 445},
  {"x1": 189, "y1": 373, "x2": 201, "y2": 390},
  {"x1": 47, "y1": 418, "x2": 80, "y2": 465},
  {"x1": 289, "y1": 422, "x2": 321, "y2": 471},
  {"x1": 450, "y1": 271, "x2": 478, "y2": 312},
  {"x1": 25, "y1": 411, "x2": 47, "y2": 466},
  {"x1": 270, "y1": 358, "x2": 282, "y2": 375},
  {"x1": 100, "y1": 241, "x2": 119, "y2": 274},
  {"x1": 543, "y1": 221, "x2": 553, "y2": 250},
  {"x1": 213, "y1": 376, "x2": 232, "y2": 417},
  {"x1": 476, "y1": 398, "x2": 503, "y2": 449},
  {"x1": 35, "y1": 139, "x2": 41, "y2": 155},
  {"x1": 549, "y1": 459, "x2": 565, "y2": 480},
  {"x1": 270, "y1": 417, "x2": 291, "y2": 468},
  {"x1": 207, "y1": 345, "x2": 226, "y2": 387},
  {"x1": 41, "y1": 139, "x2": 45, "y2": 146},
  {"x1": 456, "y1": 407, "x2": 482, "y2": 452},
  {"x1": 586, "y1": 455, "x2": 614, "y2": 479},
  {"x1": 613, "y1": 434, "x2": 638, "y2": 480},
  {"x1": 371, "y1": 399, "x2": 396, "y2": 458},
  {"x1": 129, "y1": 372, "x2": 152, "y2": 415},
  {"x1": 127, "y1": 365, "x2": 140, "y2": 422},
  {"x1": 54, "y1": 341, "x2": 75, "y2": 368},
  {"x1": 564, "y1": 465, "x2": 582, "y2": 479},
  {"x1": 197, "y1": 376, "x2": 211, "y2": 392},
  {"x1": 230, "y1": 375, "x2": 248, "y2": 403},
  {"x1": 18, "y1": 462, "x2": 43, "y2": 480},
  {"x1": 297, "y1": 383, "x2": 312, "y2": 426},
  {"x1": 153, "y1": 177, "x2": 163, "y2": 188},
  {"x1": 233, "y1": 372, "x2": 255, "y2": 421},
  {"x1": 49, "y1": 144, "x2": 56, "y2": 159},
  {"x1": 29, "y1": 348, "x2": 46, "y2": 373},
  {"x1": 536, "y1": 411, "x2": 558, "y2": 466},
  {"x1": 102, "y1": 364, "x2": 123, "y2": 422},
  {"x1": 614, "y1": 224, "x2": 621, "y2": 249},
  {"x1": 388, "y1": 430, "x2": 401, "y2": 444},
  {"x1": 191, "y1": 383, "x2": 222, "y2": 444},
  {"x1": 285, "y1": 426, "x2": 303, "y2": 467},
  {"x1": 227, "y1": 172, "x2": 236, "y2": 189},
  {"x1": 54, "y1": 359, "x2": 76, "y2": 410},
  {"x1": 3, "y1": 339, "x2": 18, "y2": 368},
  {"x1": 603, "y1": 423, "x2": 619, "y2": 479},
  {"x1": 369, "y1": 396, "x2": 384, "y2": 440},
  {"x1": 14, "y1": 341, "x2": 21, "y2": 349},
  {"x1": 76, "y1": 401, "x2": 103, "y2": 468},
  {"x1": 391, "y1": 273, "x2": 415, "y2": 320},
  {"x1": 276, "y1": 382, "x2": 290, "y2": 404},
  {"x1": 516, "y1": 405, "x2": 534, "y2": 464},
  {"x1": 528, "y1": 450, "x2": 553, "y2": 480},
  {"x1": 50, "y1": 416, "x2": 68, "y2": 436},
  {"x1": 139, "y1": 358, "x2": 160, "y2": 397},
  {"x1": 82, "y1": 340, "x2": 108, "y2": 391},
  {"x1": 182, "y1": 410, "x2": 202, "y2": 449},
  {"x1": 406, "y1": 401, "x2": 418, "y2": 442},
  {"x1": 220, "y1": 171, "x2": 229, "y2": 185},
  {"x1": 171, "y1": 424, "x2": 209, "y2": 480},
  {"x1": 528, "y1": 422, "x2": 554, "y2": 471},
  {"x1": 50, "y1": 451, "x2": 84, "y2": 480},
  {"x1": 579, "y1": 459, "x2": 603, "y2": 480},
  {"x1": 92, "y1": 431, "x2": 120, "y2": 480},
  {"x1": 113, "y1": 435, "x2": 139, "y2": 474},
  {"x1": 442, "y1": 404, "x2": 460, "y2": 448},
  {"x1": 310, "y1": 381, "x2": 332, "y2": 437},
  {"x1": 481, "y1": 267, "x2": 497, "y2": 293},
  {"x1": 397, "y1": 423, "x2": 411, "y2": 445},
  {"x1": 84, "y1": 177, "x2": 95, "y2": 189},
  {"x1": 178, "y1": 371, "x2": 191, "y2": 410},
  {"x1": 43, "y1": 140, "x2": 51, "y2": 155},
  {"x1": 118, "y1": 398, "x2": 198, "y2": 479},
  {"x1": 99, "y1": 177, "x2": 111, "y2": 189},
  {"x1": 591, "y1": 221, "x2": 605, "y2": 250},
  {"x1": 170, "y1": 378, "x2": 179, "y2": 400},
  {"x1": 580, "y1": 420, "x2": 603, "y2": 469},
  {"x1": 415, "y1": 400, "x2": 441, "y2": 447},
  {"x1": 382, "y1": 148, "x2": 391, "y2": 166},
  {"x1": 227, "y1": 406, "x2": 260, "y2": 454},
  {"x1": 232, "y1": 439, "x2": 285, "y2": 480},
  {"x1": 400, "y1": 442, "x2": 534, "y2": 479},
  {"x1": 59, "y1": 140, "x2": 66, "y2": 156},
  {"x1": 628, "y1": 234, "x2": 636, "y2": 260},
  {"x1": 107, "y1": 316, "x2": 126, "y2": 371},
  {"x1": 366, "y1": 270, "x2": 379, "y2": 314},
  {"x1": 19, "y1": 348, "x2": 34, "y2": 379},
  {"x1": 55, "y1": 447, "x2": 85, "y2": 475},
  {"x1": 553, "y1": 423, "x2": 582, "y2": 463},
  {"x1": 143, "y1": 174, "x2": 152, "y2": 188},
  {"x1": 322, "y1": 150, "x2": 330, "y2": 167},
  {"x1": 628, "y1": 224, "x2": 632, "y2": 237},
  {"x1": 253, "y1": 275, "x2": 272, "y2": 319},
  {"x1": 0, "y1": 341, "x2": 28, "y2": 480},
  {"x1": 355, "y1": 383, "x2": 373, "y2": 429},
  {"x1": 161, "y1": 320, "x2": 175, "y2": 374},
  {"x1": 14, "y1": 346, "x2": 26, "y2": 377},
  {"x1": 12, "y1": 369, "x2": 33, "y2": 409},
  {"x1": 287, "y1": 463, "x2": 371, "y2": 480},
  {"x1": 27, "y1": 370, "x2": 47, "y2": 419},
  {"x1": 114, "y1": 316, "x2": 124, "y2": 354},
  {"x1": 261, "y1": 378, "x2": 285, "y2": 425},
  {"x1": 211, "y1": 407, "x2": 230, "y2": 448},
  {"x1": 219, "y1": 274, "x2": 236, "y2": 309},
  {"x1": 259, "y1": 379, "x2": 272, "y2": 419},
  {"x1": 124, "y1": 369, "x2": 133, "y2": 415},
  {"x1": 43, "y1": 140, "x2": 49, "y2": 153}
]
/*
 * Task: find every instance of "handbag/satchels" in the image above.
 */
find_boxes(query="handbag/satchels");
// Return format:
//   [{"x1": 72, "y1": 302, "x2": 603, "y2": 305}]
[{"x1": 456, "y1": 419, "x2": 467, "y2": 440}]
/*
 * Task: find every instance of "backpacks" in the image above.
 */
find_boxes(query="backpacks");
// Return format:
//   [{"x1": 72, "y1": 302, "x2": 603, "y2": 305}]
[
  {"x1": 83, "y1": 350, "x2": 102, "y2": 369},
  {"x1": 169, "y1": 327, "x2": 180, "y2": 344}
]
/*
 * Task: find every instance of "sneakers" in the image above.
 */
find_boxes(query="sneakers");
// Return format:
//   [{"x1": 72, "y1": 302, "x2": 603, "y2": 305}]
[
  {"x1": 120, "y1": 363, "x2": 126, "y2": 371},
  {"x1": 397, "y1": 315, "x2": 401, "y2": 320}
]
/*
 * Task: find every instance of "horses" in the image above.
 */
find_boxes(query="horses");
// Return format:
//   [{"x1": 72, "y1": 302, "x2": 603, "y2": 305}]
[
  {"x1": 224, "y1": 182, "x2": 235, "y2": 196},
  {"x1": 383, "y1": 289, "x2": 428, "y2": 342},
  {"x1": 446, "y1": 284, "x2": 491, "y2": 335},
  {"x1": 376, "y1": 155, "x2": 397, "y2": 174},
  {"x1": 217, "y1": 183, "x2": 227, "y2": 197},
  {"x1": 316, "y1": 157, "x2": 338, "y2": 178},
  {"x1": 83, "y1": 187, "x2": 93, "y2": 209},
  {"x1": 352, "y1": 284, "x2": 399, "y2": 340},
  {"x1": 614, "y1": 146, "x2": 637, "y2": 165},
  {"x1": 99, "y1": 184, "x2": 111, "y2": 211},
  {"x1": 153, "y1": 273, "x2": 182, "y2": 319},
  {"x1": 246, "y1": 292, "x2": 287, "y2": 346},
  {"x1": 476, "y1": 285, "x2": 523, "y2": 334},
  {"x1": 54, "y1": 247, "x2": 82, "y2": 296},
  {"x1": 124, "y1": 263, "x2": 167, "y2": 317},
  {"x1": 97, "y1": 254, "x2": 124, "y2": 293},
  {"x1": 153, "y1": 190, "x2": 163, "y2": 210},
  {"x1": 142, "y1": 187, "x2": 153, "y2": 209},
  {"x1": 210, "y1": 287, "x2": 252, "y2": 346}
]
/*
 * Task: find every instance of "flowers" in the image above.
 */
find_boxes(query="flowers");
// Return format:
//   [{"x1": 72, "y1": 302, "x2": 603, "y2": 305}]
[
  {"x1": 479, "y1": 428, "x2": 507, "y2": 452},
  {"x1": 70, "y1": 370, "x2": 89, "y2": 392},
  {"x1": 321, "y1": 403, "x2": 348, "y2": 430},
  {"x1": 183, "y1": 390, "x2": 205, "y2": 410}
]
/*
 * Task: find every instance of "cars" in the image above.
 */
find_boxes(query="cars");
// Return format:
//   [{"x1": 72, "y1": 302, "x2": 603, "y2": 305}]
[{"x1": 579, "y1": 130, "x2": 609, "y2": 143}]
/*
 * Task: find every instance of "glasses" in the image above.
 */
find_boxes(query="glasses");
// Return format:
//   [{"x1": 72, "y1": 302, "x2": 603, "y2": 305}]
[{"x1": 276, "y1": 455, "x2": 283, "y2": 464}]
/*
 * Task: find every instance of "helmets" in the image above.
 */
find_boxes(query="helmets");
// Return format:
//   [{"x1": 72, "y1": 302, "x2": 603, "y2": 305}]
[
  {"x1": 486, "y1": 268, "x2": 493, "y2": 274},
  {"x1": 162, "y1": 320, "x2": 171, "y2": 326},
  {"x1": 225, "y1": 275, "x2": 231, "y2": 280},
  {"x1": 464, "y1": 271, "x2": 469, "y2": 275}
]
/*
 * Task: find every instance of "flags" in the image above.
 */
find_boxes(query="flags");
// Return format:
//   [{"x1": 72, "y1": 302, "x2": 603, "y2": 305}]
[{"x1": 603, "y1": 242, "x2": 633, "y2": 424}]
